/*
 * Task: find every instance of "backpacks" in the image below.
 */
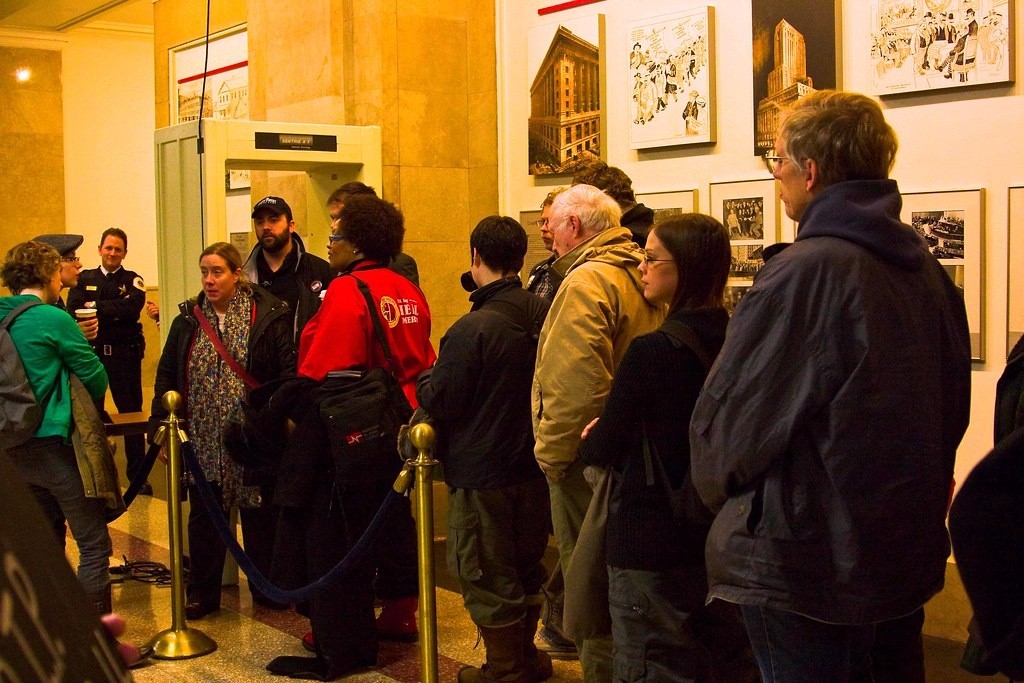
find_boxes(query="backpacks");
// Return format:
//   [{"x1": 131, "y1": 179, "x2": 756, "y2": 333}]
[
  {"x1": 0, "y1": 303, "x2": 62, "y2": 449},
  {"x1": 642, "y1": 319, "x2": 718, "y2": 546}
]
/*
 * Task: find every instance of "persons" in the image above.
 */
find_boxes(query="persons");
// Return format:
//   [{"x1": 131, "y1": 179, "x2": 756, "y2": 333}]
[
  {"x1": 533, "y1": 183, "x2": 671, "y2": 657},
  {"x1": 948, "y1": 333, "x2": 1024, "y2": 683},
  {"x1": 147, "y1": 242, "x2": 292, "y2": 620},
  {"x1": 326, "y1": 181, "x2": 420, "y2": 283},
  {"x1": 571, "y1": 161, "x2": 656, "y2": 251},
  {"x1": 298, "y1": 195, "x2": 434, "y2": 650},
  {"x1": 240, "y1": 196, "x2": 336, "y2": 329},
  {"x1": 525, "y1": 187, "x2": 571, "y2": 301},
  {"x1": 147, "y1": 301, "x2": 160, "y2": 332},
  {"x1": 64, "y1": 227, "x2": 152, "y2": 496},
  {"x1": 418, "y1": 216, "x2": 553, "y2": 683},
  {"x1": 693, "y1": 91, "x2": 971, "y2": 683},
  {"x1": 0, "y1": 241, "x2": 153, "y2": 667},
  {"x1": 583, "y1": 215, "x2": 730, "y2": 683},
  {"x1": 29, "y1": 234, "x2": 99, "y2": 339}
]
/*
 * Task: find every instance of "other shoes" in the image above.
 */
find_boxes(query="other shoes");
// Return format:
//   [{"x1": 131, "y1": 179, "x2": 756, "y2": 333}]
[
  {"x1": 130, "y1": 478, "x2": 153, "y2": 496},
  {"x1": 538, "y1": 625, "x2": 576, "y2": 649},
  {"x1": 252, "y1": 590, "x2": 289, "y2": 609},
  {"x1": 185, "y1": 601, "x2": 220, "y2": 621}
]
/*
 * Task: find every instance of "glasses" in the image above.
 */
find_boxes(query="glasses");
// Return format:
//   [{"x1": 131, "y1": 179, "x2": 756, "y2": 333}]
[
  {"x1": 60, "y1": 256, "x2": 79, "y2": 267},
  {"x1": 764, "y1": 149, "x2": 790, "y2": 174},
  {"x1": 644, "y1": 255, "x2": 675, "y2": 267},
  {"x1": 535, "y1": 219, "x2": 549, "y2": 228},
  {"x1": 329, "y1": 235, "x2": 345, "y2": 245}
]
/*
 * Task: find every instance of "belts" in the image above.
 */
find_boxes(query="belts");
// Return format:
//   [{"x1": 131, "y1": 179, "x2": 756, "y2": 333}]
[{"x1": 92, "y1": 344, "x2": 122, "y2": 356}]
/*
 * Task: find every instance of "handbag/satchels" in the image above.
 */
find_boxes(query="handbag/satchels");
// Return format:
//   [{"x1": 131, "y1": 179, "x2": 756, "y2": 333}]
[{"x1": 319, "y1": 276, "x2": 416, "y2": 488}]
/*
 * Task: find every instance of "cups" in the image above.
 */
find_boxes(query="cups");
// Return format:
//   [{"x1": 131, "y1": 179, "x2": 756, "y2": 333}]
[
  {"x1": 75, "y1": 309, "x2": 98, "y2": 324},
  {"x1": 319, "y1": 290, "x2": 327, "y2": 302}
]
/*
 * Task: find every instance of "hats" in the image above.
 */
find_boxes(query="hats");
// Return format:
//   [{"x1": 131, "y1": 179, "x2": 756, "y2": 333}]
[
  {"x1": 31, "y1": 234, "x2": 84, "y2": 256},
  {"x1": 251, "y1": 197, "x2": 292, "y2": 219}
]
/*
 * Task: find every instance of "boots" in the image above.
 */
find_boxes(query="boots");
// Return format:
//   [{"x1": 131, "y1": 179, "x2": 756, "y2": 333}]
[
  {"x1": 303, "y1": 597, "x2": 420, "y2": 654},
  {"x1": 458, "y1": 589, "x2": 551, "y2": 683},
  {"x1": 101, "y1": 616, "x2": 155, "y2": 667}
]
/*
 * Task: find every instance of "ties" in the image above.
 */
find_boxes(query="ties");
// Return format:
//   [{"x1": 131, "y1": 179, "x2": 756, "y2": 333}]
[{"x1": 106, "y1": 272, "x2": 114, "y2": 281}]
